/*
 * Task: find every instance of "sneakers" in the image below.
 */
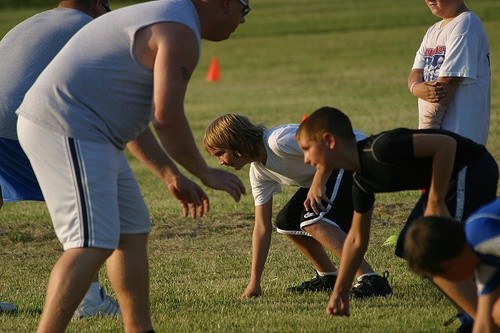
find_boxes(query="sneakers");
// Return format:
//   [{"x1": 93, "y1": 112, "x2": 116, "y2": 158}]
[
  {"x1": 349, "y1": 272, "x2": 392, "y2": 299},
  {"x1": 75, "y1": 288, "x2": 121, "y2": 317},
  {"x1": 286, "y1": 268, "x2": 337, "y2": 293}
]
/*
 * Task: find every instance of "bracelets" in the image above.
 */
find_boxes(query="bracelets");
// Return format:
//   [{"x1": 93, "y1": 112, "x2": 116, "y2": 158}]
[{"x1": 410, "y1": 81, "x2": 420, "y2": 95}]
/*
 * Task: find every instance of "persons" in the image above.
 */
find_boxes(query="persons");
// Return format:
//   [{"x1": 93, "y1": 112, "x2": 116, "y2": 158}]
[
  {"x1": 203, "y1": 112, "x2": 392, "y2": 298},
  {"x1": 16, "y1": 1, "x2": 255, "y2": 333},
  {"x1": 296, "y1": 107, "x2": 500, "y2": 333},
  {"x1": 0, "y1": 0, "x2": 127, "y2": 320},
  {"x1": 408, "y1": 0, "x2": 492, "y2": 191}
]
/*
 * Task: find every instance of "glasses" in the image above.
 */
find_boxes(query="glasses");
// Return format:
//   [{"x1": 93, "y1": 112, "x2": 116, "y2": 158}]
[{"x1": 239, "y1": 0, "x2": 250, "y2": 17}]
